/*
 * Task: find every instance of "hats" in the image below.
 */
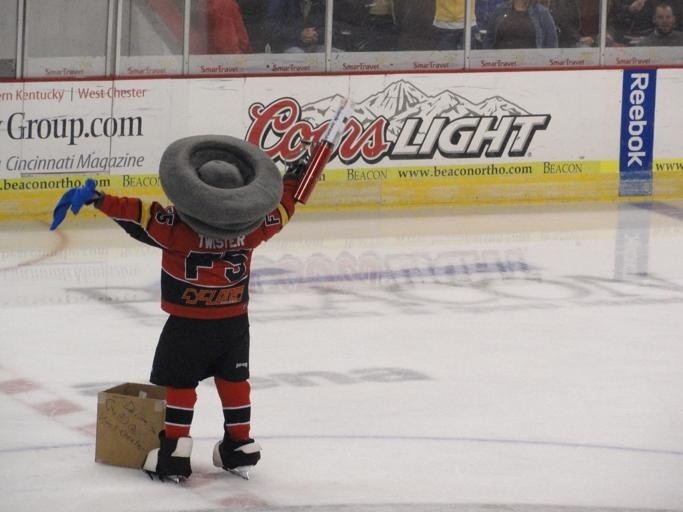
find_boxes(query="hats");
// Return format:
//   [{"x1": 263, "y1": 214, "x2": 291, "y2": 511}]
[{"x1": 157, "y1": 135, "x2": 285, "y2": 238}]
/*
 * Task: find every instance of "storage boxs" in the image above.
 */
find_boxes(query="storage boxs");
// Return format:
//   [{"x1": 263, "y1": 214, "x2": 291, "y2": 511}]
[{"x1": 95, "y1": 382, "x2": 167, "y2": 470}]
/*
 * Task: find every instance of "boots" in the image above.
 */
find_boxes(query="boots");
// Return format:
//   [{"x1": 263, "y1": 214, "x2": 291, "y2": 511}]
[
  {"x1": 213, "y1": 439, "x2": 261, "y2": 470},
  {"x1": 141, "y1": 430, "x2": 192, "y2": 478}
]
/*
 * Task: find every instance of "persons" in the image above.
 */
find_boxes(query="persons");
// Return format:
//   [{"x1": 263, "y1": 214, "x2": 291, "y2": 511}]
[
  {"x1": 51, "y1": 154, "x2": 307, "y2": 479},
  {"x1": 209, "y1": 1, "x2": 683, "y2": 53}
]
